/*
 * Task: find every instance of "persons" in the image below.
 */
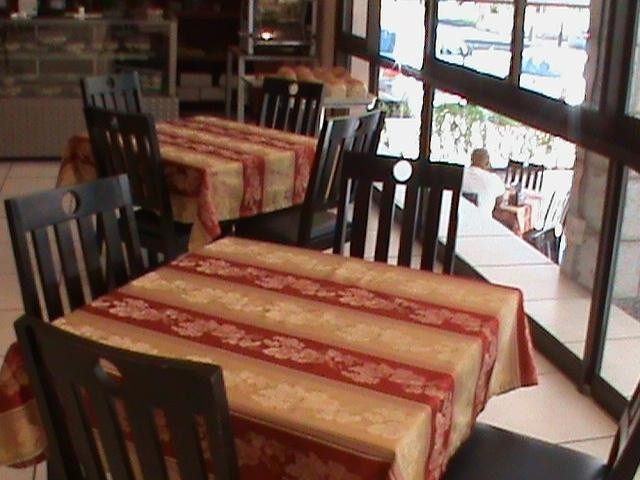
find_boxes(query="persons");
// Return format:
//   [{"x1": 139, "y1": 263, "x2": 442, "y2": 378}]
[{"x1": 458, "y1": 148, "x2": 508, "y2": 221}]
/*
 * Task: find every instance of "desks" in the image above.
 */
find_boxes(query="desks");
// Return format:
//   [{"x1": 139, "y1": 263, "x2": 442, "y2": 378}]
[{"x1": 227, "y1": 45, "x2": 320, "y2": 124}]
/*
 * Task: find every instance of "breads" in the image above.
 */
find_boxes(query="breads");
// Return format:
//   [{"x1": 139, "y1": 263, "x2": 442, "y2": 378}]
[{"x1": 277, "y1": 65, "x2": 368, "y2": 98}]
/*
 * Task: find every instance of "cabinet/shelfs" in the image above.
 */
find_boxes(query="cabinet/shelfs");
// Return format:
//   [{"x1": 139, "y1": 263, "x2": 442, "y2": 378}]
[{"x1": 0, "y1": 18, "x2": 179, "y2": 158}]
